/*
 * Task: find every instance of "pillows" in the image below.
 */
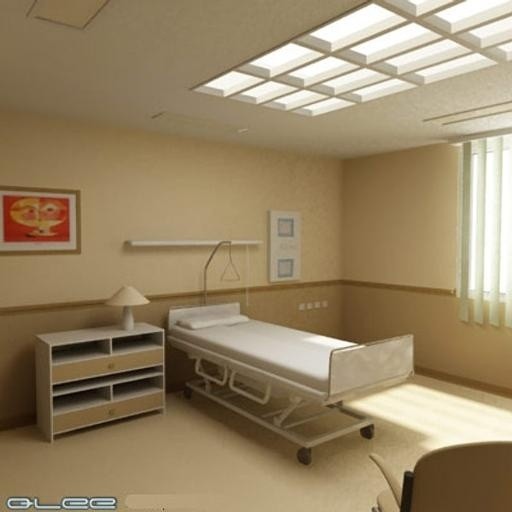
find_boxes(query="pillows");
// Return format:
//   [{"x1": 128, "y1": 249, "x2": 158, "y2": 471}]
[{"x1": 175, "y1": 308, "x2": 249, "y2": 329}]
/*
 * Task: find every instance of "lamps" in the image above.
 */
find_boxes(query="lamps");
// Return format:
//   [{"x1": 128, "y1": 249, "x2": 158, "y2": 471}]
[
  {"x1": 104, "y1": 286, "x2": 152, "y2": 329},
  {"x1": 200, "y1": 239, "x2": 242, "y2": 310}
]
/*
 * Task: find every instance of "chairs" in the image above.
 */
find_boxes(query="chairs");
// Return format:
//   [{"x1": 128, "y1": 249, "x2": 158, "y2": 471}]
[{"x1": 370, "y1": 443, "x2": 512, "y2": 511}]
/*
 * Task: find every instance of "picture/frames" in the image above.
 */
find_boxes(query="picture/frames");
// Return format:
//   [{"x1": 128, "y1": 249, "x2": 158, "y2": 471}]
[{"x1": 1, "y1": 185, "x2": 82, "y2": 256}]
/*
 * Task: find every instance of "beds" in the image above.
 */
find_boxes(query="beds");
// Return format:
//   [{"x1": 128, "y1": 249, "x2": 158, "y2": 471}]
[{"x1": 169, "y1": 302, "x2": 416, "y2": 464}]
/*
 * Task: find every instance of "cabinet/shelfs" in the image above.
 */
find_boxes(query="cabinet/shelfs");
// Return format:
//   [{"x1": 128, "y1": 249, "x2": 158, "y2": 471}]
[{"x1": 31, "y1": 319, "x2": 167, "y2": 441}]
